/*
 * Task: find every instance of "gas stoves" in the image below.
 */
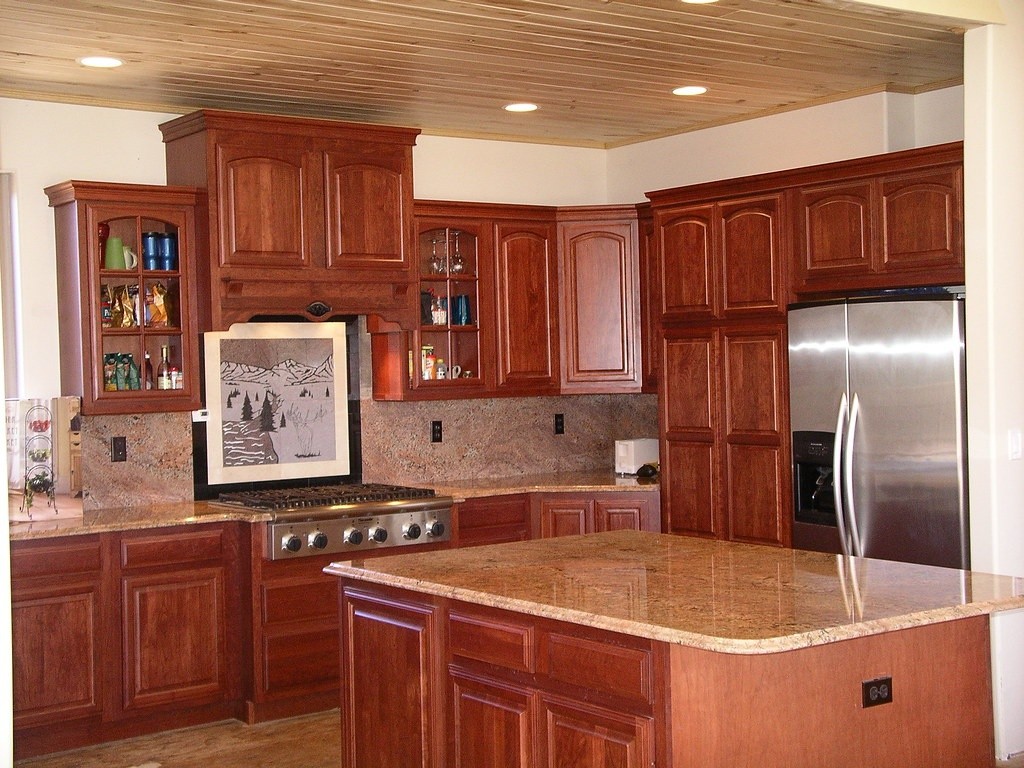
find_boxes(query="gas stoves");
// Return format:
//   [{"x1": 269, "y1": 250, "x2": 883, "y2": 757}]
[{"x1": 208, "y1": 483, "x2": 454, "y2": 561}]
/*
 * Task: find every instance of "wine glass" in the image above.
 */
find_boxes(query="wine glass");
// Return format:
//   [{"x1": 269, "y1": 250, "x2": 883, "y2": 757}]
[{"x1": 428, "y1": 231, "x2": 468, "y2": 274}]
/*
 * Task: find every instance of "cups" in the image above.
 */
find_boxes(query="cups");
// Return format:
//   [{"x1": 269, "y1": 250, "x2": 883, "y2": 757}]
[
  {"x1": 105, "y1": 238, "x2": 125, "y2": 270},
  {"x1": 121, "y1": 245, "x2": 138, "y2": 270},
  {"x1": 445, "y1": 366, "x2": 461, "y2": 380},
  {"x1": 455, "y1": 296, "x2": 471, "y2": 323},
  {"x1": 141, "y1": 231, "x2": 176, "y2": 271}
]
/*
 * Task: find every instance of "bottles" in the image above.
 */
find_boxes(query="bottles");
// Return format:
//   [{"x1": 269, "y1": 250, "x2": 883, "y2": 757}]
[
  {"x1": 138, "y1": 351, "x2": 153, "y2": 391},
  {"x1": 431, "y1": 298, "x2": 447, "y2": 326},
  {"x1": 422, "y1": 346, "x2": 446, "y2": 380},
  {"x1": 157, "y1": 343, "x2": 183, "y2": 390}
]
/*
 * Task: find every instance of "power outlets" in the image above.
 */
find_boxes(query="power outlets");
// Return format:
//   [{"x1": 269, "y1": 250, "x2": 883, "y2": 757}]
[
  {"x1": 430, "y1": 420, "x2": 444, "y2": 442},
  {"x1": 861, "y1": 676, "x2": 893, "y2": 709},
  {"x1": 554, "y1": 413, "x2": 565, "y2": 435},
  {"x1": 111, "y1": 436, "x2": 127, "y2": 462}
]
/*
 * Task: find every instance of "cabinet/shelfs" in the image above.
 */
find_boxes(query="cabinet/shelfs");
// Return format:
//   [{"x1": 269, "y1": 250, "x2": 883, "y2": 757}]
[
  {"x1": 110, "y1": 521, "x2": 238, "y2": 741},
  {"x1": 556, "y1": 205, "x2": 643, "y2": 394},
  {"x1": 370, "y1": 199, "x2": 490, "y2": 401},
  {"x1": 10, "y1": 533, "x2": 110, "y2": 760},
  {"x1": 43, "y1": 180, "x2": 202, "y2": 416},
  {"x1": 157, "y1": 109, "x2": 421, "y2": 333},
  {"x1": 535, "y1": 491, "x2": 661, "y2": 538},
  {"x1": 635, "y1": 202, "x2": 658, "y2": 393},
  {"x1": 787, "y1": 137, "x2": 966, "y2": 304},
  {"x1": 657, "y1": 323, "x2": 792, "y2": 548},
  {"x1": 643, "y1": 169, "x2": 786, "y2": 326},
  {"x1": 339, "y1": 575, "x2": 995, "y2": 768},
  {"x1": 488, "y1": 202, "x2": 558, "y2": 398}
]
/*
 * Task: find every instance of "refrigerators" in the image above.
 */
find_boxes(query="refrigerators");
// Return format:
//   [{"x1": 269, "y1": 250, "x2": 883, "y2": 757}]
[{"x1": 786, "y1": 289, "x2": 969, "y2": 574}]
